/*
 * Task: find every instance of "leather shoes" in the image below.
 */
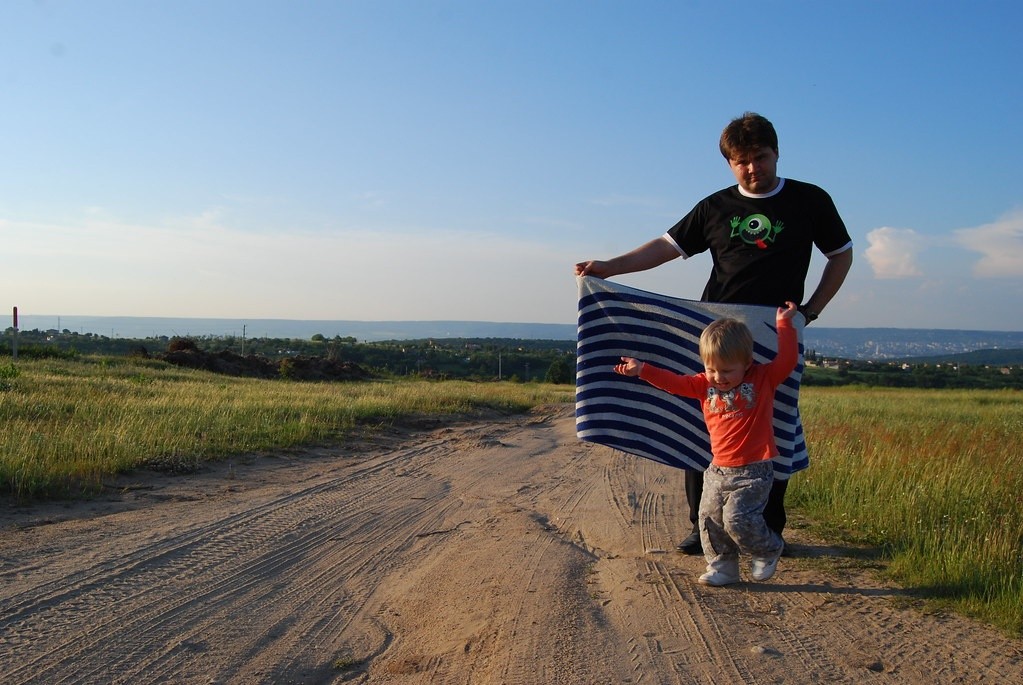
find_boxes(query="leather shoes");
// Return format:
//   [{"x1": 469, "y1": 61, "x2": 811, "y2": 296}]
[{"x1": 676, "y1": 531, "x2": 700, "y2": 551}]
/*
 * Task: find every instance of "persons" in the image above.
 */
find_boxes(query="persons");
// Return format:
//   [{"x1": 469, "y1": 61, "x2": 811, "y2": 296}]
[
  {"x1": 613, "y1": 302, "x2": 799, "y2": 588},
  {"x1": 573, "y1": 111, "x2": 854, "y2": 554}
]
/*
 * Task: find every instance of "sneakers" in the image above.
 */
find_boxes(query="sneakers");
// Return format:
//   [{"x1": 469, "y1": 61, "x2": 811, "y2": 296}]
[
  {"x1": 752, "y1": 540, "x2": 785, "y2": 581},
  {"x1": 698, "y1": 565, "x2": 740, "y2": 587}
]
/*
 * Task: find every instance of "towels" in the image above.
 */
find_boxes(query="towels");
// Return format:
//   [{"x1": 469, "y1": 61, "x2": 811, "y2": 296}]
[{"x1": 574, "y1": 276, "x2": 809, "y2": 482}]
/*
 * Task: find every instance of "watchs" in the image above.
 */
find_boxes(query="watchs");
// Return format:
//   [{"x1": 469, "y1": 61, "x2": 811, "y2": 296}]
[{"x1": 804, "y1": 304, "x2": 818, "y2": 322}]
